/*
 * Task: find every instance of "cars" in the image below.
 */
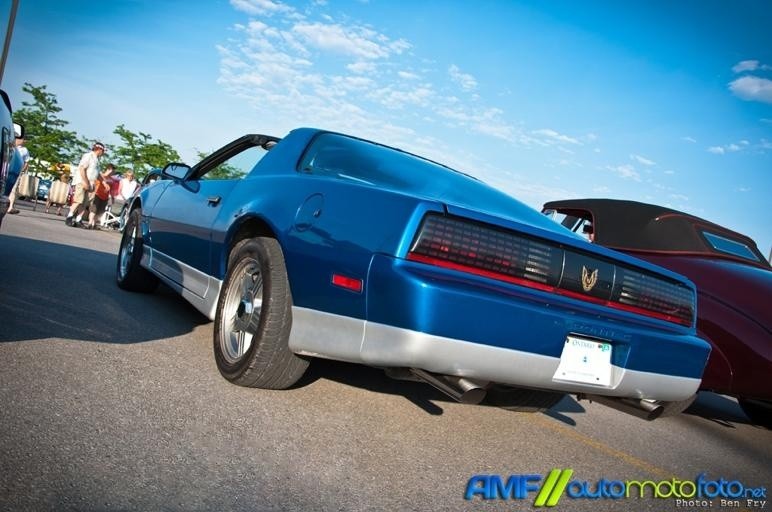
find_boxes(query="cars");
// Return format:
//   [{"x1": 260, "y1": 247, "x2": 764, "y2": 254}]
[
  {"x1": 538, "y1": 199, "x2": 772, "y2": 428},
  {"x1": 0, "y1": 87, "x2": 23, "y2": 225},
  {"x1": 116, "y1": 128, "x2": 713, "y2": 420}
]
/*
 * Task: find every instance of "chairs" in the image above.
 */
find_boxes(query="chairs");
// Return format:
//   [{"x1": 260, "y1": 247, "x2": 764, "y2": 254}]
[
  {"x1": 45, "y1": 181, "x2": 70, "y2": 216},
  {"x1": 13, "y1": 175, "x2": 40, "y2": 211}
]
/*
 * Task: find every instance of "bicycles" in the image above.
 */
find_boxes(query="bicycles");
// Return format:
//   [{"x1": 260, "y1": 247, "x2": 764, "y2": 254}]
[{"x1": 98, "y1": 203, "x2": 128, "y2": 232}]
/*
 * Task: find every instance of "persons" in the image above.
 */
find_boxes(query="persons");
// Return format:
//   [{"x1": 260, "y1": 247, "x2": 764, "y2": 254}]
[
  {"x1": 64, "y1": 141, "x2": 112, "y2": 230},
  {"x1": 4, "y1": 134, "x2": 30, "y2": 216},
  {"x1": 44, "y1": 172, "x2": 69, "y2": 215},
  {"x1": 108, "y1": 169, "x2": 141, "y2": 230},
  {"x1": 84, "y1": 164, "x2": 117, "y2": 230}
]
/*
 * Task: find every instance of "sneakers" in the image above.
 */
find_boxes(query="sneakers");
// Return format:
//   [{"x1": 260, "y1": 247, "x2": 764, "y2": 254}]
[
  {"x1": 65, "y1": 216, "x2": 101, "y2": 230},
  {"x1": 6, "y1": 208, "x2": 19, "y2": 214}
]
